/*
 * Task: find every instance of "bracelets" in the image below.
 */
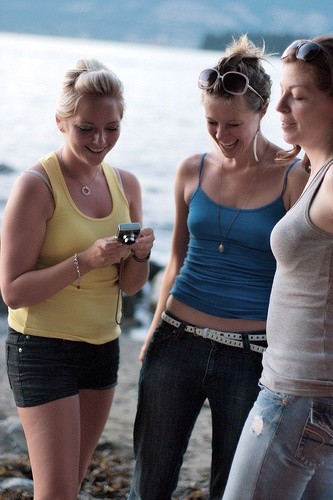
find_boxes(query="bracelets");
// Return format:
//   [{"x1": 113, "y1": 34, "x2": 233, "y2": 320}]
[
  {"x1": 74, "y1": 252, "x2": 81, "y2": 290},
  {"x1": 132, "y1": 250, "x2": 151, "y2": 263}
]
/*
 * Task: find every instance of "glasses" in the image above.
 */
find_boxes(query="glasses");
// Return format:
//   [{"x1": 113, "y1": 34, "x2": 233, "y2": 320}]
[
  {"x1": 199, "y1": 69, "x2": 264, "y2": 103},
  {"x1": 281, "y1": 40, "x2": 333, "y2": 74}
]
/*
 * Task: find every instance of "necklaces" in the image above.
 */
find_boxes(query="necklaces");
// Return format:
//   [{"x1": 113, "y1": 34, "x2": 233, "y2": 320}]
[
  {"x1": 61, "y1": 147, "x2": 100, "y2": 197},
  {"x1": 217, "y1": 141, "x2": 270, "y2": 253}
]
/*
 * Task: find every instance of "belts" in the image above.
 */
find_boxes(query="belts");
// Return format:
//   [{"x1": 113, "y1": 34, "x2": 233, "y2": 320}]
[{"x1": 160, "y1": 311, "x2": 266, "y2": 353}]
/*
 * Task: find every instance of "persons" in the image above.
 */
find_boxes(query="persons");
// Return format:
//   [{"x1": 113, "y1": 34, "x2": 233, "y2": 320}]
[
  {"x1": 0, "y1": 57, "x2": 156, "y2": 500},
  {"x1": 221, "y1": 35, "x2": 333, "y2": 500},
  {"x1": 127, "y1": 32, "x2": 310, "y2": 500}
]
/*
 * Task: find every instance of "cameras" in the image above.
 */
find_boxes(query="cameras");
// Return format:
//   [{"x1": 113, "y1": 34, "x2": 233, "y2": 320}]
[{"x1": 114, "y1": 223, "x2": 141, "y2": 245}]
